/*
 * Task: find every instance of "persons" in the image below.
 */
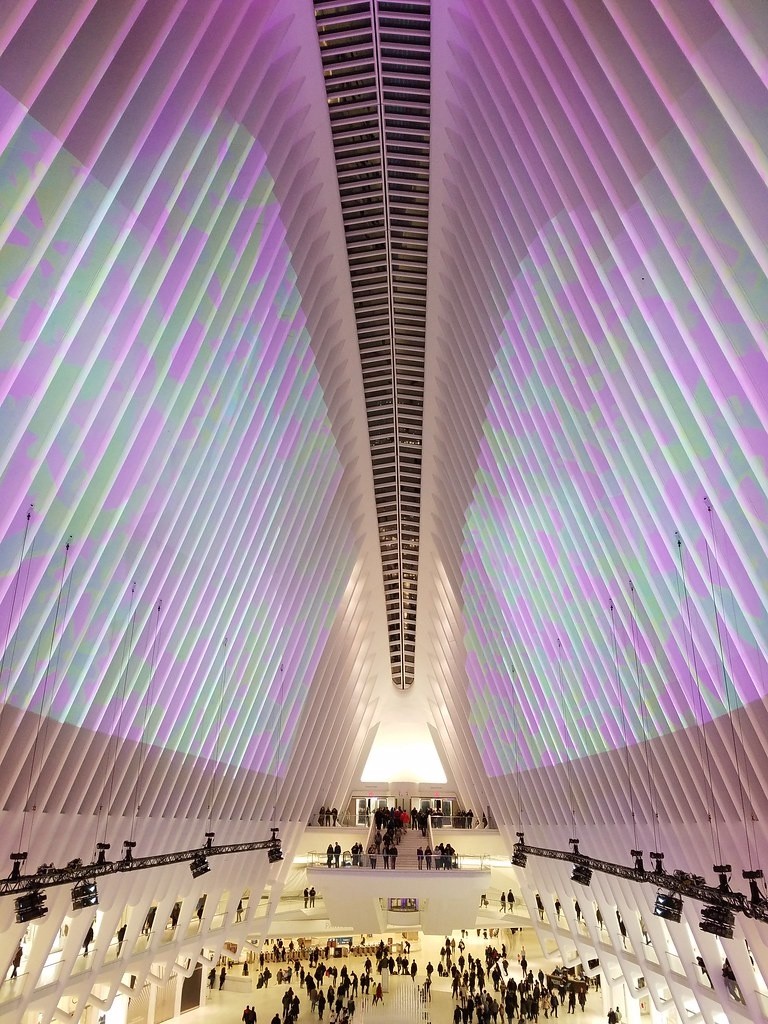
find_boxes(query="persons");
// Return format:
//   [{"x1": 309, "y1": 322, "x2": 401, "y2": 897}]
[{"x1": 1, "y1": 806, "x2": 767, "y2": 1024}]
